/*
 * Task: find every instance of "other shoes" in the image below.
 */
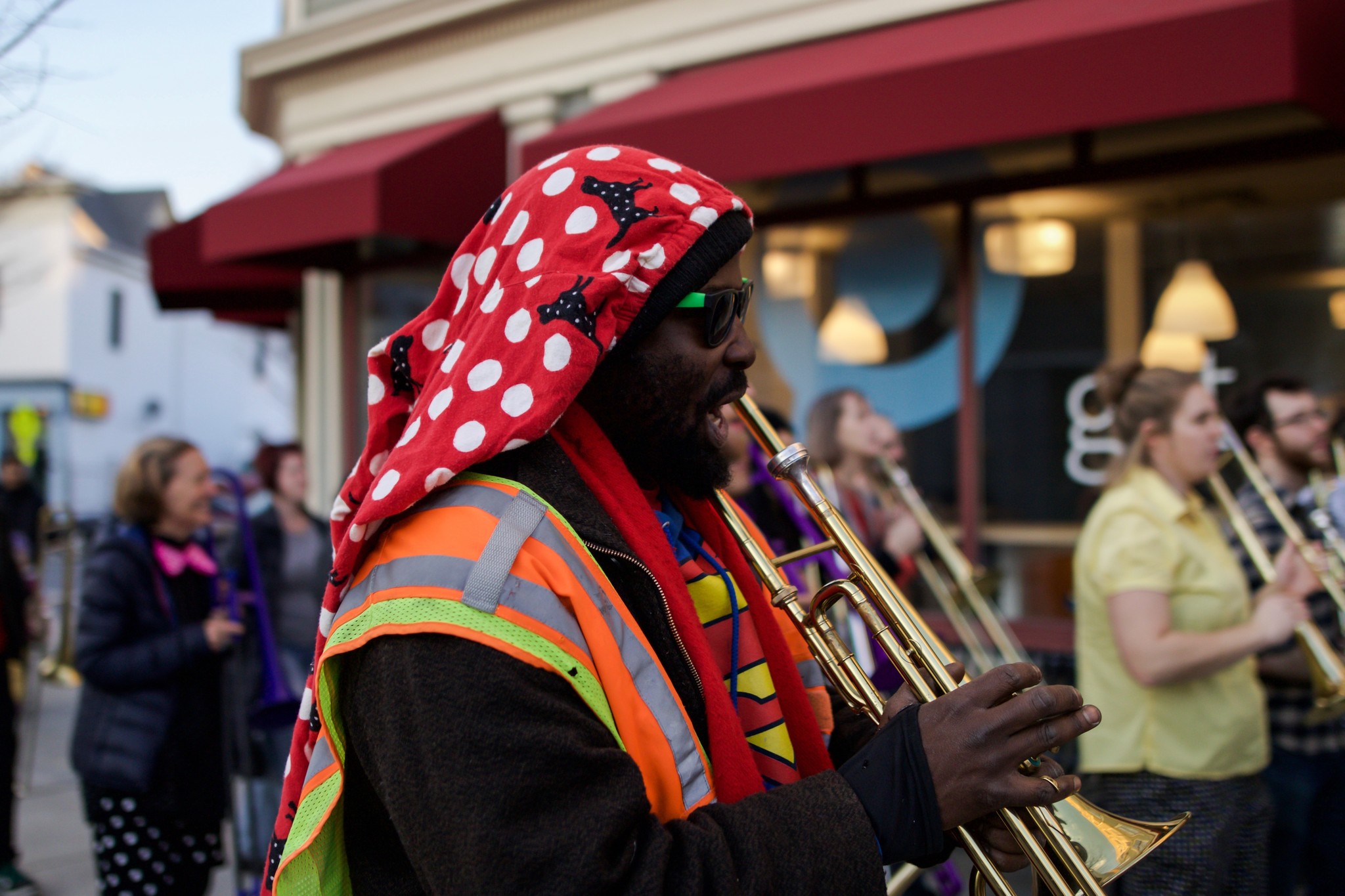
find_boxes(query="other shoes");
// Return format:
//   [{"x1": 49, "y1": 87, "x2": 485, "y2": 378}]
[{"x1": 0, "y1": 871, "x2": 39, "y2": 896}]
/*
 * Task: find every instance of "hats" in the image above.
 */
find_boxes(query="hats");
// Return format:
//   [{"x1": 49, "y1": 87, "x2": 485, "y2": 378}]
[{"x1": 618, "y1": 214, "x2": 755, "y2": 358}]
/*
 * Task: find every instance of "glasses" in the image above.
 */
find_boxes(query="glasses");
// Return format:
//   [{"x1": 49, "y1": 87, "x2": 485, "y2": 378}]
[{"x1": 676, "y1": 279, "x2": 754, "y2": 351}]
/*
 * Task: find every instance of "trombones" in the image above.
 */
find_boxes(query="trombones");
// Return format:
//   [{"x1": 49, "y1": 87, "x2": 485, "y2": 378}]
[
  {"x1": 869, "y1": 448, "x2": 1068, "y2": 762},
  {"x1": 1203, "y1": 414, "x2": 1345, "y2": 727},
  {"x1": 200, "y1": 465, "x2": 304, "y2": 896},
  {"x1": 704, "y1": 387, "x2": 1196, "y2": 896},
  {"x1": 20, "y1": 507, "x2": 85, "y2": 802}
]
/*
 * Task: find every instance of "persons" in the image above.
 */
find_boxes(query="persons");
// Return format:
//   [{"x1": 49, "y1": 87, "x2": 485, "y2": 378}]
[
  {"x1": 1221, "y1": 374, "x2": 1345, "y2": 896},
  {"x1": 1070, "y1": 355, "x2": 1329, "y2": 896},
  {"x1": 69, "y1": 435, "x2": 244, "y2": 896},
  {"x1": 260, "y1": 144, "x2": 1103, "y2": 896},
  {"x1": 226, "y1": 443, "x2": 332, "y2": 896},
  {"x1": 710, "y1": 384, "x2": 969, "y2": 896},
  {"x1": 0, "y1": 442, "x2": 49, "y2": 896}
]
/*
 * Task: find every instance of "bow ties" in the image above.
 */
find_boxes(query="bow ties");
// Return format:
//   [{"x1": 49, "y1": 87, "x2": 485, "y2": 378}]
[{"x1": 156, "y1": 543, "x2": 216, "y2": 575}]
